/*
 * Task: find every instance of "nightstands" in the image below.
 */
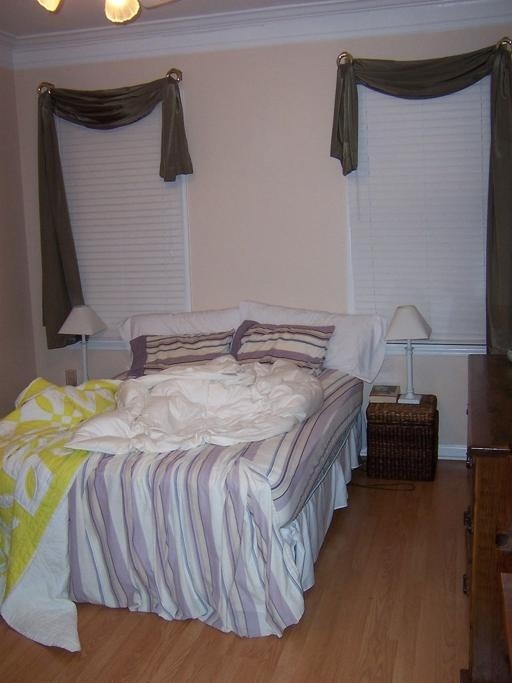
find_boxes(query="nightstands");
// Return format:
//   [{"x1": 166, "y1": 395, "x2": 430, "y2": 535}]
[{"x1": 361, "y1": 392, "x2": 438, "y2": 480}]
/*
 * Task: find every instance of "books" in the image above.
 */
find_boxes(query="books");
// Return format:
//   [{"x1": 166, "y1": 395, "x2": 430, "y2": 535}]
[{"x1": 368, "y1": 384, "x2": 400, "y2": 403}]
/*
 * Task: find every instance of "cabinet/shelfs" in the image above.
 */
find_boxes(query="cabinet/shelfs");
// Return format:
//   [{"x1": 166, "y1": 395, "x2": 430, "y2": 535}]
[{"x1": 463, "y1": 354, "x2": 512, "y2": 683}]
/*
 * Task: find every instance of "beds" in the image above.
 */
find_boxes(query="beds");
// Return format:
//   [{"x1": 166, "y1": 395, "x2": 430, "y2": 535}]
[{"x1": 2, "y1": 370, "x2": 381, "y2": 623}]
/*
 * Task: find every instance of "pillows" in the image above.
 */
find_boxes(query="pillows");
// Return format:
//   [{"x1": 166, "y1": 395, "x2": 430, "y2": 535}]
[{"x1": 113, "y1": 308, "x2": 382, "y2": 384}]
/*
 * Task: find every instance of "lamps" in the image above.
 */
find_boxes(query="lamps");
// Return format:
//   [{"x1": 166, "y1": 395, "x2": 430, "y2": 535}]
[
  {"x1": 104, "y1": 0, "x2": 141, "y2": 24},
  {"x1": 55, "y1": 303, "x2": 107, "y2": 387},
  {"x1": 386, "y1": 307, "x2": 432, "y2": 406},
  {"x1": 36, "y1": 0, "x2": 62, "y2": 13}
]
